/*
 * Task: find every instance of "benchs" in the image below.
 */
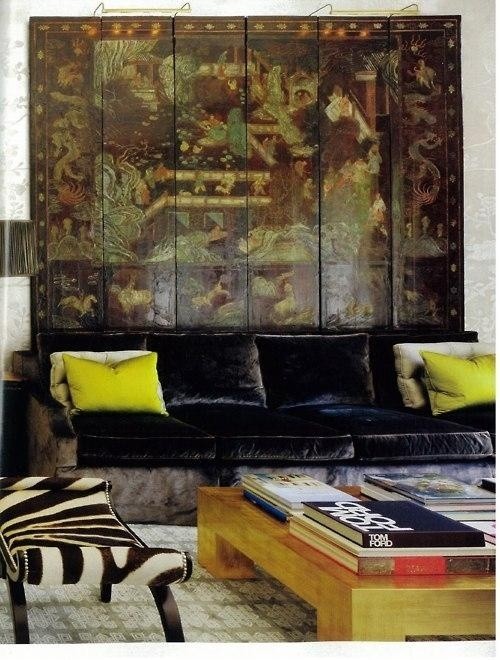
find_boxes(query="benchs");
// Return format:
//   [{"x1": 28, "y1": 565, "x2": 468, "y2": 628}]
[{"x1": 0, "y1": 477, "x2": 193, "y2": 642}]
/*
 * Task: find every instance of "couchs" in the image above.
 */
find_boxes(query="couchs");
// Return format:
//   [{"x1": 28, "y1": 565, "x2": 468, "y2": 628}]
[{"x1": 26, "y1": 326, "x2": 495, "y2": 525}]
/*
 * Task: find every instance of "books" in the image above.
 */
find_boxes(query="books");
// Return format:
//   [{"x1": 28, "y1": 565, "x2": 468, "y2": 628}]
[{"x1": 238, "y1": 470, "x2": 495, "y2": 578}]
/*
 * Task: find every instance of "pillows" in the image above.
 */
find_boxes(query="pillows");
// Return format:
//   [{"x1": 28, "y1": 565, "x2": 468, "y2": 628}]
[
  {"x1": 393, "y1": 340, "x2": 495, "y2": 417},
  {"x1": 47, "y1": 347, "x2": 170, "y2": 418}
]
